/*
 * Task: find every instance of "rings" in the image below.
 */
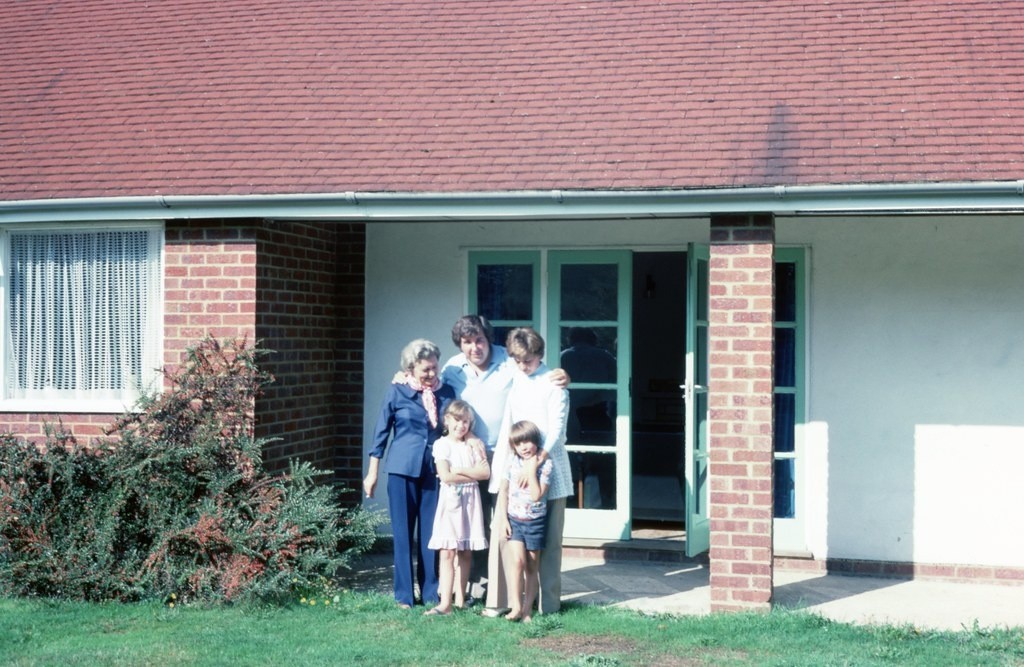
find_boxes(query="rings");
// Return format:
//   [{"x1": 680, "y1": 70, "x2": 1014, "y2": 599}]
[{"x1": 365, "y1": 495, "x2": 370, "y2": 499}]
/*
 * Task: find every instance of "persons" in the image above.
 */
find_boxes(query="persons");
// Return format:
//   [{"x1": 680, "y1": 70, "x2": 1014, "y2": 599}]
[
  {"x1": 439, "y1": 314, "x2": 570, "y2": 607},
  {"x1": 559, "y1": 326, "x2": 616, "y2": 509},
  {"x1": 362, "y1": 338, "x2": 457, "y2": 611},
  {"x1": 499, "y1": 419, "x2": 554, "y2": 626},
  {"x1": 422, "y1": 399, "x2": 491, "y2": 617},
  {"x1": 482, "y1": 326, "x2": 575, "y2": 617}
]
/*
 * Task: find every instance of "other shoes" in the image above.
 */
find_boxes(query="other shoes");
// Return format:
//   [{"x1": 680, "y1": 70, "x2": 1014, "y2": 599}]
[
  {"x1": 396, "y1": 602, "x2": 410, "y2": 609},
  {"x1": 481, "y1": 607, "x2": 510, "y2": 617},
  {"x1": 463, "y1": 595, "x2": 475, "y2": 607}
]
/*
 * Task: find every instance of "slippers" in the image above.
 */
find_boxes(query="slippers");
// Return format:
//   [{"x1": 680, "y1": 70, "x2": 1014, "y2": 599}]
[{"x1": 422, "y1": 608, "x2": 451, "y2": 616}]
